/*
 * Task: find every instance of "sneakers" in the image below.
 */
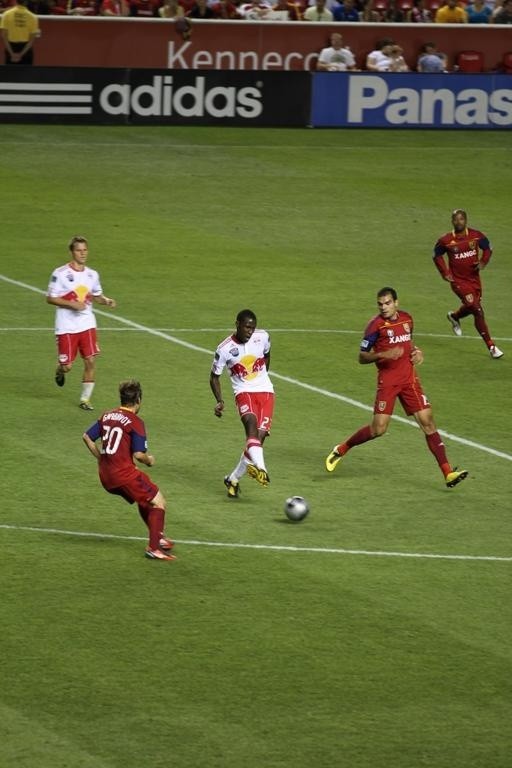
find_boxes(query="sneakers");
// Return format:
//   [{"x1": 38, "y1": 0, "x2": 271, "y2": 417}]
[
  {"x1": 145, "y1": 545, "x2": 177, "y2": 560},
  {"x1": 325, "y1": 445, "x2": 345, "y2": 472},
  {"x1": 55, "y1": 369, "x2": 64, "y2": 387},
  {"x1": 446, "y1": 470, "x2": 468, "y2": 489},
  {"x1": 447, "y1": 310, "x2": 462, "y2": 337},
  {"x1": 158, "y1": 533, "x2": 173, "y2": 550},
  {"x1": 224, "y1": 475, "x2": 239, "y2": 498},
  {"x1": 246, "y1": 463, "x2": 271, "y2": 488},
  {"x1": 79, "y1": 400, "x2": 94, "y2": 411},
  {"x1": 490, "y1": 344, "x2": 504, "y2": 359}
]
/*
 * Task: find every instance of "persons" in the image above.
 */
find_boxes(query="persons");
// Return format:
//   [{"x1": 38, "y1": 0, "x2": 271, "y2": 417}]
[
  {"x1": 433, "y1": 209, "x2": 503, "y2": 359},
  {"x1": 83, "y1": 378, "x2": 177, "y2": 561},
  {"x1": 209, "y1": 309, "x2": 275, "y2": 499},
  {"x1": 46, "y1": 235, "x2": 116, "y2": 410},
  {"x1": 325, "y1": 287, "x2": 468, "y2": 489}
]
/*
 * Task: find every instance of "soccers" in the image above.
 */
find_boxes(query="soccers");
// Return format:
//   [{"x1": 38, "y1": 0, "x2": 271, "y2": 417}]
[{"x1": 285, "y1": 496, "x2": 308, "y2": 520}]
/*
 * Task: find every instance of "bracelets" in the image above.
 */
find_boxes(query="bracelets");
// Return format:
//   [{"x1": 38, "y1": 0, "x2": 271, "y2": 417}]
[{"x1": 217, "y1": 400, "x2": 224, "y2": 403}]
